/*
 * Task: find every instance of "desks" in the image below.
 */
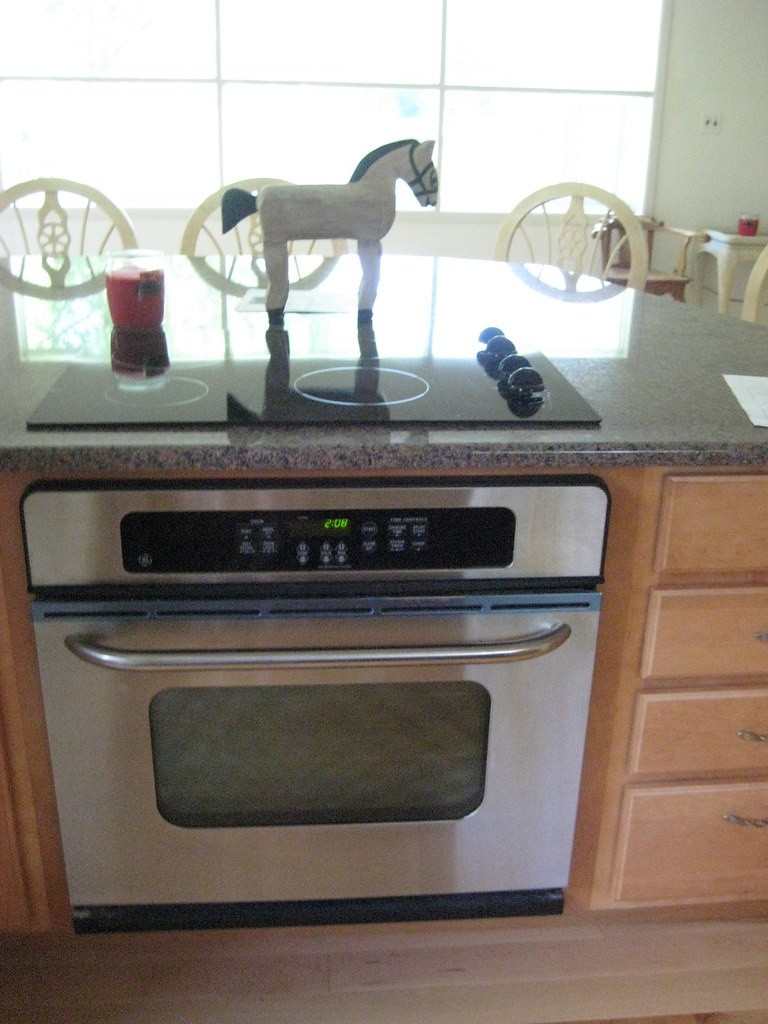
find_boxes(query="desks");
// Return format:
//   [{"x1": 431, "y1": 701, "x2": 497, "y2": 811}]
[
  {"x1": 0, "y1": 249, "x2": 768, "y2": 466},
  {"x1": 694, "y1": 226, "x2": 768, "y2": 314}
]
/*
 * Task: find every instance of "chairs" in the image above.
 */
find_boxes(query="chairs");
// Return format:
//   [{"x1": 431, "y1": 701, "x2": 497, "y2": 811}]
[
  {"x1": 592, "y1": 215, "x2": 690, "y2": 303},
  {"x1": 180, "y1": 178, "x2": 349, "y2": 256},
  {"x1": 493, "y1": 182, "x2": 649, "y2": 291},
  {"x1": 740, "y1": 244, "x2": 768, "y2": 327},
  {"x1": 0, "y1": 178, "x2": 137, "y2": 256}
]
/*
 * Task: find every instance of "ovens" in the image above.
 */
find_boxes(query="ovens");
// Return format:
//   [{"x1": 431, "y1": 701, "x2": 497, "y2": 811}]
[{"x1": 22, "y1": 476, "x2": 614, "y2": 935}]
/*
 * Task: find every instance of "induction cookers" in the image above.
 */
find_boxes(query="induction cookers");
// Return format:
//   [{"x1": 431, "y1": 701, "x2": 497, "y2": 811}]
[{"x1": 25, "y1": 325, "x2": 606, "y2": 429}]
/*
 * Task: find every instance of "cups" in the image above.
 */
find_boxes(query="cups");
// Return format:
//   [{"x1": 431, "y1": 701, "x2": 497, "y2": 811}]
[
  {"x1": 105, "y1": 249, "x2": 163, "y2": 329},
  {"x1": 738, "y1": 213, "x2": 760, "y2": 237}
]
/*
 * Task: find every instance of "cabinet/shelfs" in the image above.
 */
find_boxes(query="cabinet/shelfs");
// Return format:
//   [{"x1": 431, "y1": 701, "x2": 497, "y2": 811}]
[{"x1": 589, "y1": 468, "x2": 768, "y2": 925}]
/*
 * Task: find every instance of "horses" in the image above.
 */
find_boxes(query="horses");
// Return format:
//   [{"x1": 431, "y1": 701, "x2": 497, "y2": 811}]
[{"x1": 221, "y1": 139, "x2": 438, "y2": 327}]
